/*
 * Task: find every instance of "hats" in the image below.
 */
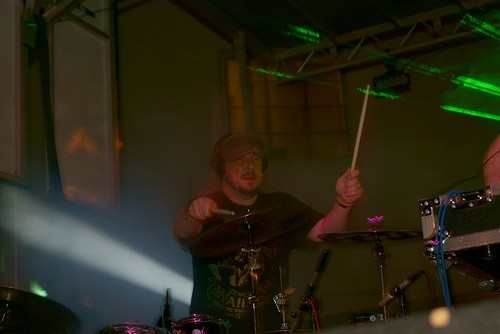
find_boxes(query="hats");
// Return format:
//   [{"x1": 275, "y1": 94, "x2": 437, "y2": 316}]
[{"x1": 220, "y1": 134, "x2": 264, "y2": 162}]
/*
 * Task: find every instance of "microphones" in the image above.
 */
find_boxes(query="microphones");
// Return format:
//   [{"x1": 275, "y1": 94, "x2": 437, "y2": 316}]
[
  {"x1": 379, "y1": 271, "x2": 422, "y2": 307},
  {"x1": 157, "y1": 291, "x2": 171, "y2": 328}
]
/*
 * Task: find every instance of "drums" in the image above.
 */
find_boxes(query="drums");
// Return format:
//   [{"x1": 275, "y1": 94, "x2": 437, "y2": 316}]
[
  {"x1": 166, "y1": 313, "x2": 229, "y2": 334},
  {"x1": 93, "y1": 320, "x2": 164, "y2": 334}
]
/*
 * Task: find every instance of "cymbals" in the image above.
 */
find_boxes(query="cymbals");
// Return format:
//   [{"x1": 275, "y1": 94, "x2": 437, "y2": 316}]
[
  {"x1": 0, "y1": 283, "x2": 82, "y2": 334},
  {"x1": 317, "y1": 228, "x2": 422, "y2": 244},
  {"x1": 189, "y1": 204, "x2": 312, "y2": 258}
]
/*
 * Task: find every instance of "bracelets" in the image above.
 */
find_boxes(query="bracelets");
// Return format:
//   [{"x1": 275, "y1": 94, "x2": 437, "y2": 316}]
[{"x1": 336, "y1": 196, "x2": 353, "y2": 208}]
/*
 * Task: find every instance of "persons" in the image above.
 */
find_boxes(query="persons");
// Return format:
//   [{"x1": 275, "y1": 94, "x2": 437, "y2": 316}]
[{"x1": 172, "y1": 131, "x2": 365, "y2": 334}]
